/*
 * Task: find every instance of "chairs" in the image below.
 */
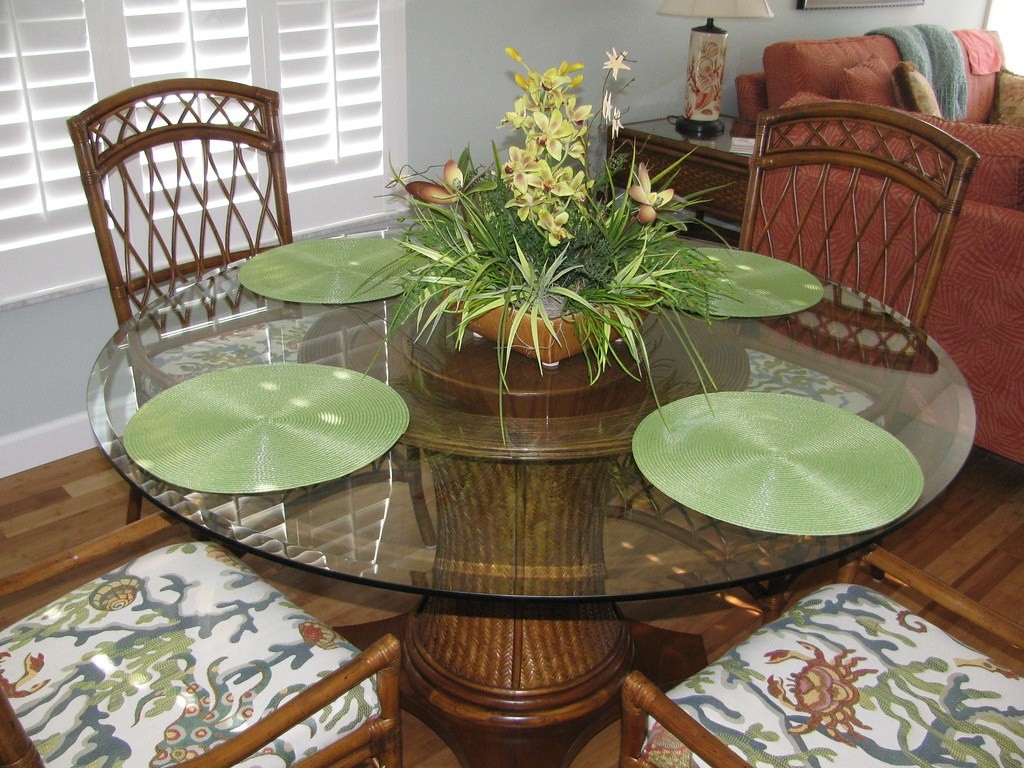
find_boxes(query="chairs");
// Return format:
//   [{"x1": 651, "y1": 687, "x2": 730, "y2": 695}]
[
  {"x1": 0, "y1": 494, "x2": 404, "y2": 768},
  {"x1": 66, "y1": 77, "x2": 437, "y2": 565},
  {"x1": 601, "y1": 101, "x2": 980, "y2": 625},
  {"x1": 619, "y1": 538, "x2": 1024, "y2": 768}
]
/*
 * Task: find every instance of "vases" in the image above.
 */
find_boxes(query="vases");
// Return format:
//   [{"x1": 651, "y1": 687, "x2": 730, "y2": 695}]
[{"x1": 444, "y1": 283, "x2": 661, "y2": 370}]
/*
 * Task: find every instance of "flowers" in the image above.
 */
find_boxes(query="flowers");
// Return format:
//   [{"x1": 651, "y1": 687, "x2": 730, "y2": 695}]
[{"x1": 345, "y1": 45, "x2": 746, "y2": 447}]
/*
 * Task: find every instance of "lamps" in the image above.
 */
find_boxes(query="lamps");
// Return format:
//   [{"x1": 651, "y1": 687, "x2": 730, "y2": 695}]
[{"x1": 657, "y1": 0, "x2": 774, "y2": 136}]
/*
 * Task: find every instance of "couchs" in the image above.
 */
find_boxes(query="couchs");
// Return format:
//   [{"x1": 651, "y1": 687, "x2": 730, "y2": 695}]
[{"x1": 735, "y1": 30, "x2": 1024, "y2": 462}]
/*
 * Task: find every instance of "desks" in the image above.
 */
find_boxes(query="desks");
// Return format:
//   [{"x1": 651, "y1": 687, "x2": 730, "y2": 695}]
[
  {"x1": 608, "y1": 112, "x2": 756, "y2": 247},
  {"x1": 85, "y1": 223, "x2": 976, "y2": 768}
]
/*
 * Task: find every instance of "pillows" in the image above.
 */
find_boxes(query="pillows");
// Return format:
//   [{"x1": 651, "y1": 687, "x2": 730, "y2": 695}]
[
  {"x1": 988, "y1": 67, "x2": 1024, "y2": 128},
  {"x1": 838, "y1": 52, "x2": 902, "y2": 109},
  {"x1": 892, "y1": 60, "x2": 941, "y2": 117}
]
